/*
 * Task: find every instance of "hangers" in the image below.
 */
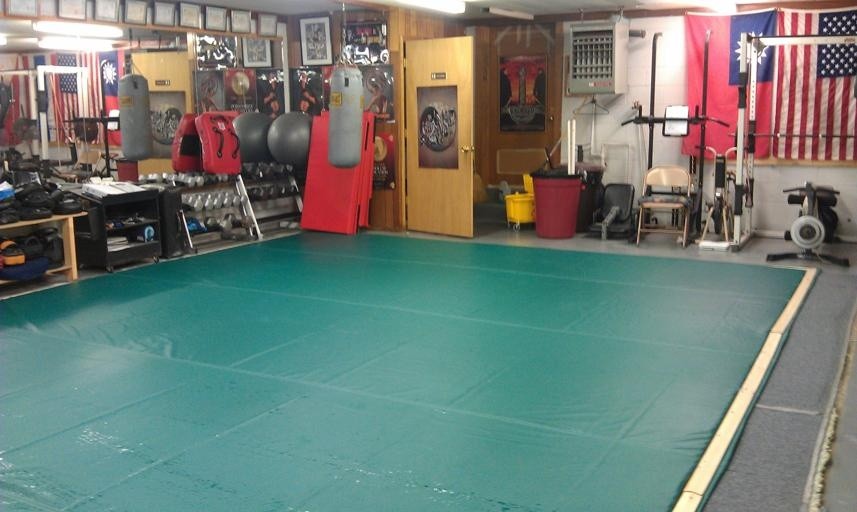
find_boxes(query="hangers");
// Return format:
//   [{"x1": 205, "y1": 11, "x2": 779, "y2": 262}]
[{"x1": 571, "y1": 93, "x2": 611, "y2": 117}]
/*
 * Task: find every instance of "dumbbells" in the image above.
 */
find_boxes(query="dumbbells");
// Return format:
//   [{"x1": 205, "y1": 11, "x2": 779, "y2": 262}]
[
  {"x1": 137, "y1": 169, "x2": 166, "y2": 184},
  {"x1": 166, "y1": 162, "x2": 298, "y2": 233}
]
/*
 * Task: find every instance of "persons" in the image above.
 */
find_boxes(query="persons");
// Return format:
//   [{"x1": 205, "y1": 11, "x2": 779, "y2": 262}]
[
  {"x1": 362, "y1": 71, "x2": 387, "y2": 114},
  {"x1": 263, "y1": 72, "x2": 283, "y2": 115},
  {"x1": 199, "y1": 75, "x2": 218, "y2": 112},
  {"x1": 296, "y1": 72, "x2": 320, "y2": 115}
]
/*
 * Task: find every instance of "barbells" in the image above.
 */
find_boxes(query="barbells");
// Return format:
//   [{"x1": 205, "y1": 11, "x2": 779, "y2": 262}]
[{"x1": 791, "y1": 185, "x2": 825, "y2": 249}]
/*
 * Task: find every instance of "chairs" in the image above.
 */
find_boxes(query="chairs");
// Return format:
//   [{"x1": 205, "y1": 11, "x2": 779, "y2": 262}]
[
  {"x1": 634, "y1": 164, "x2": 691, "y2": 247},
  {"x1": 588, "y1": 184, "x2": 640, "y2": 240},
  {"x1": 59, "y1": 148, "x2": 101, "y2": 184}
]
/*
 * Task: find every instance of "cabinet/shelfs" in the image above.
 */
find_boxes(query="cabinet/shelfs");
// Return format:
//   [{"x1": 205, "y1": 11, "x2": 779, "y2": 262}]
[
  {"x1": 71, "y1": 188, "x2": 163, "y2": 272},
  {"x1": 0, "y1": 210, "x2": 85, "y2": 285}
]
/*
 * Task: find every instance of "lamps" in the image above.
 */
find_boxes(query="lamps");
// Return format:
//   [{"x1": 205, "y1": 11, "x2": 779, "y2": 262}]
[
  {"x1": 32, "y1": 20, "x2": 123, "y2": 52},
  {"x1": 483, "y1": 7, "x2": 535, "y2": 23}
]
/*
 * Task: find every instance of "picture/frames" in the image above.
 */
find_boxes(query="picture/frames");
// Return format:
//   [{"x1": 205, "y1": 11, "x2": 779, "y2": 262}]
[
  {"x1": 125, "y1": 1, "x2": 146, "y2": 24},
  {"x1": 154, "y1": 3, "x2": 175, "y2": 26},
  {"x1": 231, "y1": 10, "x2": 252, "y2": 34},
  {"x1": 205, "y1": 7, "x2": 226, "y2": 32},
  {"x1": 258, "y1": 13, "x2": 277, "y2": 36},
  {"x1": 8, "y1": 1, "x2": 37, "y2": 17},
  {"x1": 242, "y1": 38, "x2": 272, "y2": 69},
  {"x1": 194, "y1": 30, "x2": 237, "y2": 69},
  {"x1": 297, "y1": 14, "x2": 333, "y2": 67},
  {"x1": 179, "y1": 3, "x2": 200, "y2": 29},
  {"x1": 94, "y1": 2, "x2": 120, "y2": 23},
  {"x1": 59, "y1": 2, "x2": 87, "y2": 19}
]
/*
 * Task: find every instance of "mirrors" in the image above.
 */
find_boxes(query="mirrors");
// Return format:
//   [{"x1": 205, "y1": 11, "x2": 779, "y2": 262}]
[{"x1": 0, "y1": 13, "x2": 285, "y2": 185}]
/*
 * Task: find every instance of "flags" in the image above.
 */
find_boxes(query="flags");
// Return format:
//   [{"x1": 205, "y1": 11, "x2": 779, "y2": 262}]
[
  {"x1": 683, "y1": 8, "x2": 775, "y2": 162},
  {"x1": 772, "y1": 5, "x2": 857, "y2": 161},
  {"x1": 0, "y1": 49, "x2": 125, "y2": 146}
]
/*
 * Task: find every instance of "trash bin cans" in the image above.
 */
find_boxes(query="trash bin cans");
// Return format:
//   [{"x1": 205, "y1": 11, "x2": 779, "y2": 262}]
[
  {"x1": 529, "y1": 164, "x2": 605, "y2": 239},
  {"x1": 115, "y1": 159, "x2": 138, "y2": 181}
]
